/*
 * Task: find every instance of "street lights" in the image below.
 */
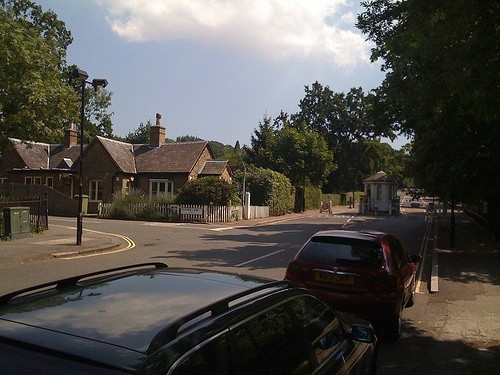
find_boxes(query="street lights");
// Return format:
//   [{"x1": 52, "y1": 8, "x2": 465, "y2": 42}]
[{"x1": 71, "y1": 68, "x2": 109, "y2": 246}]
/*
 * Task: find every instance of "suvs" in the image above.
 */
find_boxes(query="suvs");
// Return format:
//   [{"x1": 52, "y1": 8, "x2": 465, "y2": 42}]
[{"x1": 0, "y1": 261, "x2": 378, "y2": 375}]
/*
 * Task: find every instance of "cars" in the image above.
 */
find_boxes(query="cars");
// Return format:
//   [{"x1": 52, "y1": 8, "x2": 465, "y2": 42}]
[{"x1": 284, "y1": 230, "x2": 423, "y2": 342}]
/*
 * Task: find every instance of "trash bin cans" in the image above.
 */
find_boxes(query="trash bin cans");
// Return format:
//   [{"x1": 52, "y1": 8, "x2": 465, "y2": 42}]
[
  {"x1": 78, "y1": 194, "x2": 89, "y2": 214},
  {"x1": 340, "y1": 194, "x2": 346, "y2": 204}
]
